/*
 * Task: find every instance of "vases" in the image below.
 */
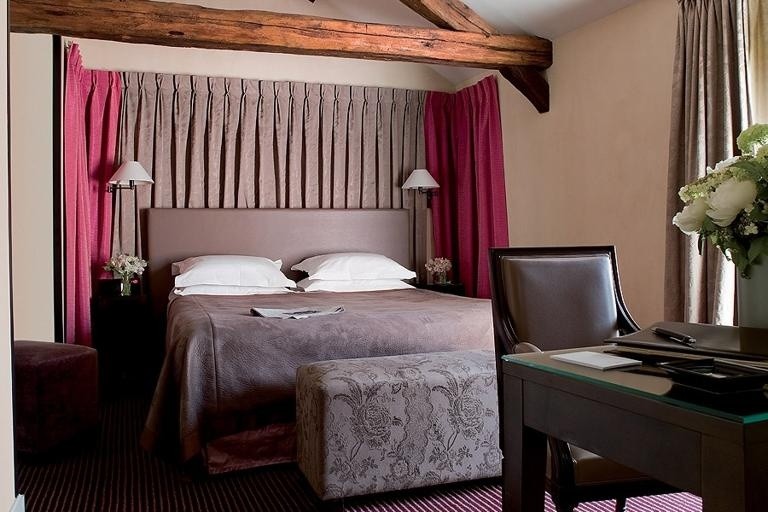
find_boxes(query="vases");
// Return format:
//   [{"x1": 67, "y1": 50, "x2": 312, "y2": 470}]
[
  {"x1": 119, "y1": 275, "x2": 133, "y2": 297},
  {"x1": 432, "y1": 270, "x2": 449, "y2": 285},
  {"x1": 730, "y1": 232, "x2": 767, "y2": 334}
]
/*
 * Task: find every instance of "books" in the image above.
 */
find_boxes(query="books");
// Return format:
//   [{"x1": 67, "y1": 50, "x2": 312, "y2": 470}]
[{"x1": 551, "y1": 350, "x2": 642, "y2": 371}]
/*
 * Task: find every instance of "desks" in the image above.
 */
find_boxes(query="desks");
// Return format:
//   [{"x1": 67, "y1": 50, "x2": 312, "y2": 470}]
[{"x1": 496, "y1": 338, "x2": 768, "y2": 511}]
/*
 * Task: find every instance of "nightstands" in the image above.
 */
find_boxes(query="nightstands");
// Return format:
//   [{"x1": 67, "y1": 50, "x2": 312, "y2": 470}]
[
  {"x1": 408, "y1": 279, "x2": 468, "y2": 297},
  {"x1": 87, "y1": 293, "x2": 163, "y2": 398}
]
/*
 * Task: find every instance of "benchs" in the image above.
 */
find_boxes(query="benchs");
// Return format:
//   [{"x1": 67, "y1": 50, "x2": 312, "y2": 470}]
[{"x1": 286, "y1": 349, "x2": 504, "y2": 504}]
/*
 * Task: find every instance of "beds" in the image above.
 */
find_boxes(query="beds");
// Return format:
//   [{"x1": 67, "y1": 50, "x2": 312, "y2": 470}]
[{"x1": 139, "y1": 205, "x2": 498, "y2": 484}]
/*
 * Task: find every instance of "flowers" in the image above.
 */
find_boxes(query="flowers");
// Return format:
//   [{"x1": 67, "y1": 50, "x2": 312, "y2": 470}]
[
  {"x1": 102, "y1": 254, "x2": 152, "y2": 281},
  {"x1": 425, "y1": 256, "x2": 454, "y2": 273},
  {"x1": 669, "y1": 116, "x2": 768, "y2": 273}
]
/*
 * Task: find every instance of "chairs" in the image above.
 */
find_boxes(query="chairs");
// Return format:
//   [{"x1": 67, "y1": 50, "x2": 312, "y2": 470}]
[{"x1": 486, "y1": 238, "x2": 692, "y2": 512}]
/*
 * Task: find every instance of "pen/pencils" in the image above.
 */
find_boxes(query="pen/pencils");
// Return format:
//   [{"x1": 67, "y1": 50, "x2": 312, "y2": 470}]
[{"x1": 651, "y1": 325, "x2": 697, "y2": 349}]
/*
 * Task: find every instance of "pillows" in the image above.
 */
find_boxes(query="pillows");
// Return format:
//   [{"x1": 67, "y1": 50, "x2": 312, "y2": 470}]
[
  {"x1": 173, "y1": 279, "x2": 294, "y2": 298},
  {"x1": 169, "y1": 253, "x2": 299, "y2": 289},
  {"x1": 290, "y1": 249, "x2": 420, "y2": 282},
  {"x1": 296, "y1": 272, "x2": 416, "y2": 294}
]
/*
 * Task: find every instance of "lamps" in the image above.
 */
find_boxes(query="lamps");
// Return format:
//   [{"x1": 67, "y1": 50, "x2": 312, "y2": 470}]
[
  {"x1": 401, "y1": 168, "x2": 443, "y2": 199},
  {"x1": 106, "y1": 160, "x2": 157, "y2": 193}
]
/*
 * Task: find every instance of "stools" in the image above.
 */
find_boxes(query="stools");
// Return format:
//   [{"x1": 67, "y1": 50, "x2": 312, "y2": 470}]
[{"x1": 13, "y1": 337, "x2": 104, "y2": 461}]
